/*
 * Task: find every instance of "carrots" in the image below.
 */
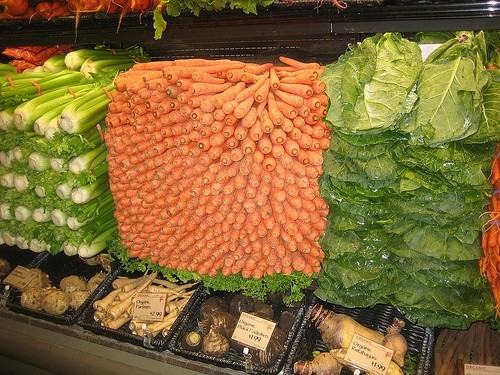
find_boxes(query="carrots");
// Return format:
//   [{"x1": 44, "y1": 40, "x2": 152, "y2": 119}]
[{"x1": 102, "y1": 56, "x2": 333, "y2": 280}]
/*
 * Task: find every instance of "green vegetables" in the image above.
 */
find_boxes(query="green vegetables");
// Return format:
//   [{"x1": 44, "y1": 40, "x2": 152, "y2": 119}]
[
  {"x1": 0, "y1": 43, "x2": 152, "y2": 258},
  {"x1": 320, "y1": 30, "x2": 500, "y2": 330}
]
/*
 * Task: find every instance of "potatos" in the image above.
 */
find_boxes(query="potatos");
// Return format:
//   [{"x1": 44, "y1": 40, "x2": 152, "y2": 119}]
[{"x1": 20, "y1": 267, "x2": 107, "y2": 316}]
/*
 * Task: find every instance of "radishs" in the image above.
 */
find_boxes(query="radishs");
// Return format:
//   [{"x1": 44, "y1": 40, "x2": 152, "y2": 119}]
[
  {"x1": 293, "y1": 303, "x2": 409, "y2": 375},
  {"x1": 92, "y1": 272, "x2": 199, "y2": 339}
]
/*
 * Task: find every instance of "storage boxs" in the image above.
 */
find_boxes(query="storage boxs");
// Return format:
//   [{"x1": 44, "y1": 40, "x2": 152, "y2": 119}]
[{"x1": 0, "y1": 243, "x2": 433, "y2": 375}]
